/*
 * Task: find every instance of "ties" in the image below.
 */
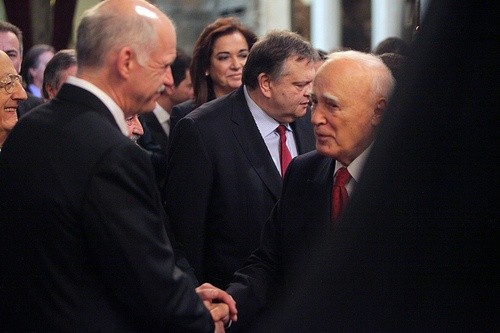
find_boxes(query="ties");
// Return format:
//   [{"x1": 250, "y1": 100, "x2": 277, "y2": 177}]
[
  {"x1": 273, "y1": 125, "x2": 295, "y2": 181},
  {"x1": 331, "y1": 167, "x2": 352, "y2": 226}
]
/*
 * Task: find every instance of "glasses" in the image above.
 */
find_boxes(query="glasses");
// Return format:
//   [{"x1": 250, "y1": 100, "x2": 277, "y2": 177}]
[{"x1": 0, "y1": 73, "x2": 23, "y2": 94}]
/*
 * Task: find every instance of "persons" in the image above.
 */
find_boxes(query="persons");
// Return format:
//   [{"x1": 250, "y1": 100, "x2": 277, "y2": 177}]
[
  {"x1": 0, "y1": 21, "x2": 78, "y2": 151},
  {"x1": 0, "y1": 0, "x2": 237, "y2": 333},
  {"x1": 276, "y1": 0, "x2": 500, "y2": 333},
  {"x1": 125, "y1": 18, "x2": 329, "y2": 292},
  {"x1": 209, "y1": 49, "x2": 396, "y2": 333}
]
